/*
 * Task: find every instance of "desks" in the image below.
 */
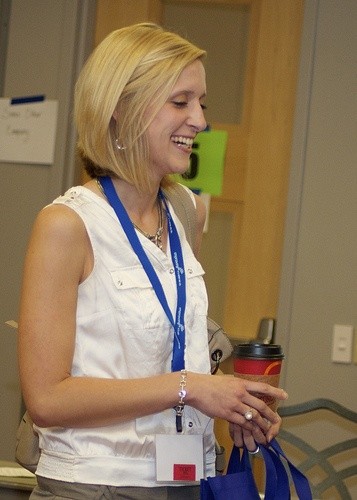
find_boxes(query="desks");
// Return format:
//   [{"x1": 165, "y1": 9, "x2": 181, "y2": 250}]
[{"x1": 0, "y1": 459, "x2": 38, "y2": 491}]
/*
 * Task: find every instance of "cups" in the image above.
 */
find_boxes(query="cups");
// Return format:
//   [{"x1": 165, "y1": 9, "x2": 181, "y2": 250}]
[{"x1": 231, "y1": 343, "x2": 285, "y2": 418}]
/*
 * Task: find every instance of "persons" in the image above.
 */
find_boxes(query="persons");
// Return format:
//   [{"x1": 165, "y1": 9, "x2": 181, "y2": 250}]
[{"x1": 19, "y1": 23, "x2": 288, "y2": 500}]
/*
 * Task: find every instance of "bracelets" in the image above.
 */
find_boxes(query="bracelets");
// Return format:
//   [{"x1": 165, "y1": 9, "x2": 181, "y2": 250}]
[{"x1": 176, "y1": 370, "x2": 187, "y2": 416}]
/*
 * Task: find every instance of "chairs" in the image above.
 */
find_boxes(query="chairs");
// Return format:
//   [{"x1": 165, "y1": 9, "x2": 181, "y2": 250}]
[{"x1": 249, "y1": 399, "x2": 357, "y2": 500}]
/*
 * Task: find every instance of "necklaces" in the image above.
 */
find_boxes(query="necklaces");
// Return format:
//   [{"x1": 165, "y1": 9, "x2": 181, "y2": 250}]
[{"x1": 95, "y1": 178, "x2": 165, "y2": 250}]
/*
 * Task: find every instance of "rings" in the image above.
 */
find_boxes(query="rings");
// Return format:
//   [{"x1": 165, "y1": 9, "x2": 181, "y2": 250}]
[
  {"x1": 244, "y1": 407, "x2": 253, "y2": 420},
  {"x1": 249, "y1": 445, "x2": 260, "y2": 455}
]
